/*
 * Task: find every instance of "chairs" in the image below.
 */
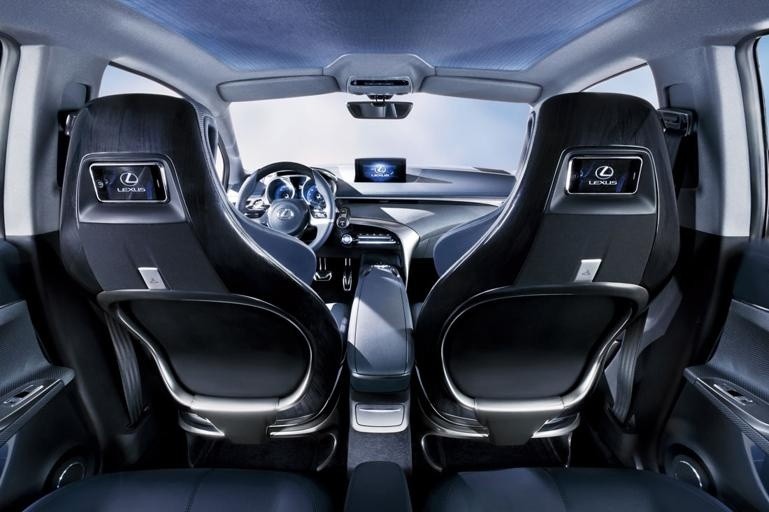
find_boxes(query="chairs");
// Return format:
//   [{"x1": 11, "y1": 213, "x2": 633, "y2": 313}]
[
  {"x1": 52, "y1": 89, "x2": 346, "y2": 472},
  {"x1": 410, "y1": 89, "x2": 685, "y2": 476}
]
